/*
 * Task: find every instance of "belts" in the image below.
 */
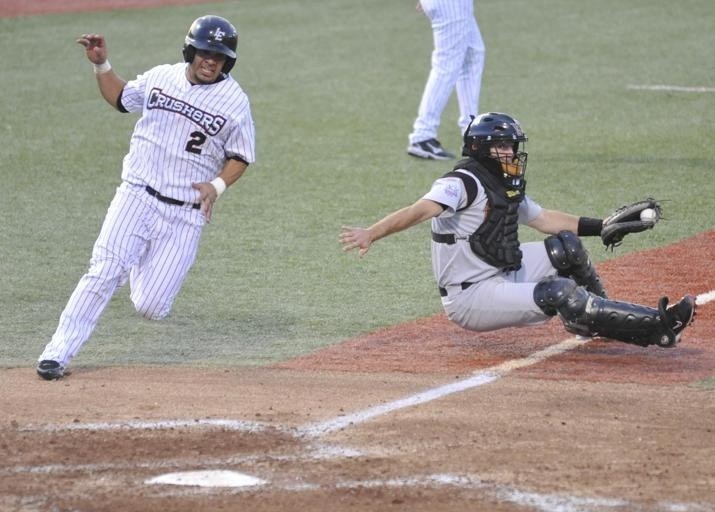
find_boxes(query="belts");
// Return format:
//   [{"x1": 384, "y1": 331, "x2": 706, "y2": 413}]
[{"x1": 146, "y1": 183, "x2": 201, "y2": 210}]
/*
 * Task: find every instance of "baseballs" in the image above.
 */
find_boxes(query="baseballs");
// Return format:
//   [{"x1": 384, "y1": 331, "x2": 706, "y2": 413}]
[{"x1": 640, "y1": 208, "x2": 656, "y2": 221}]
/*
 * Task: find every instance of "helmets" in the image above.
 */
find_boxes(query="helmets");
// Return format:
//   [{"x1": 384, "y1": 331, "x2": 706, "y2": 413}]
[
  {"x1": 461, "y1": 110, "x2": 528, "y2": 189},
  {"x1": 182, "y1": 14, "x2": 239, "y2": 75}
]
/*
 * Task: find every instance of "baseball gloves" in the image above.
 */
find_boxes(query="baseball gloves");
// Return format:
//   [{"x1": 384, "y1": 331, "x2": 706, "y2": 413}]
[{"x1": 601, "y1": 198, "x2": 672, "y2": 252}]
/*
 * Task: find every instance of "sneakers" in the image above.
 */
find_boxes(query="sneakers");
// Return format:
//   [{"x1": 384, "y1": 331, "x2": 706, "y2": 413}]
[
  {"x1": 407, "y1": 138, "x2": 458, "y2": 161},
  {"x1": 37, "y1": 360, "x2": 67, "y2": 378},
  {"x1": 665, "y1": 292, "x2": 697, "y2": 346}
]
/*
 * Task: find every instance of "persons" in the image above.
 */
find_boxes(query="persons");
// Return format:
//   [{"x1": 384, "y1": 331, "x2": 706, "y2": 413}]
[
  {"x1": 33, "y1": 13, "x2": 256, "y2": 382},
  {"x1": 405, "y1": 1, "x2": 490, "y2": 164},
  {"x1": 336, "y1": 110, "x2": 700, "y2": 349}
]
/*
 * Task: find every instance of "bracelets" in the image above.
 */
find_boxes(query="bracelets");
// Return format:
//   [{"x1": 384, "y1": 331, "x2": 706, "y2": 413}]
[
  {"x1": 93, "y1": 60, "x2": 112, "y2": 76},
  {"x1": 209, "y1": 176, "x2": 228, "y2": 203}
]
